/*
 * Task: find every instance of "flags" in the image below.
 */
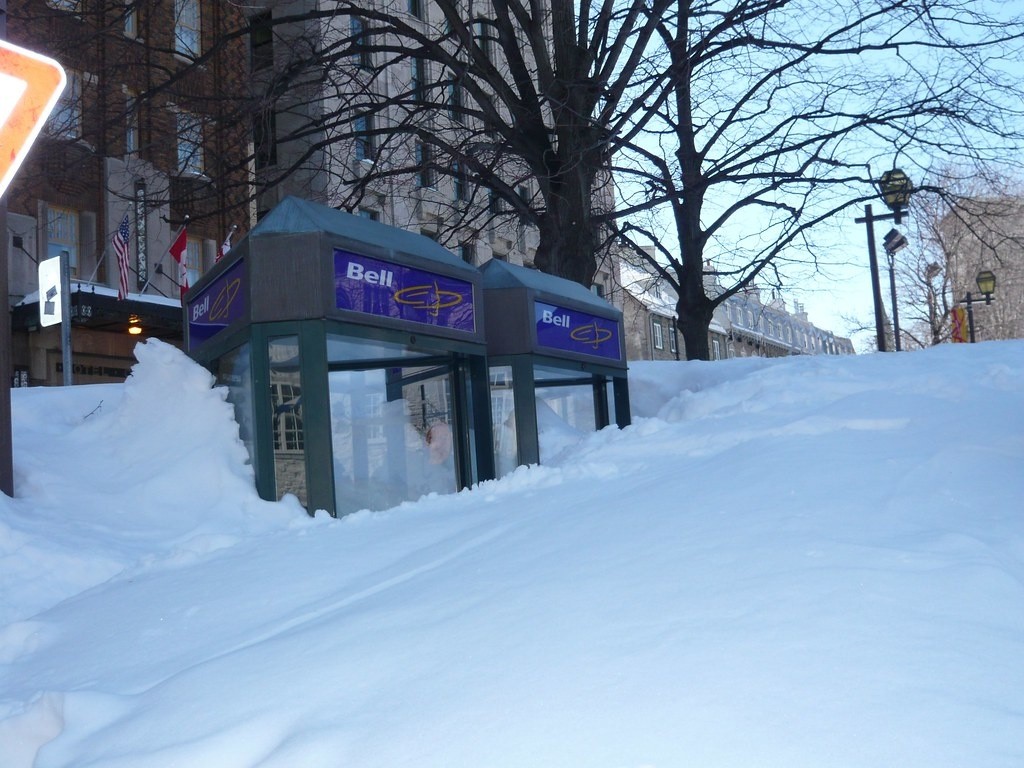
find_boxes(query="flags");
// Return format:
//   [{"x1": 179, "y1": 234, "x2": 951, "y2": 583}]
[
  {"x1": 111, "y1": 214, "x2": 131, "y2": 301},
  {"x1": 215, "y1": 240, "x2": 232, "y2": 265},
  {"x1": 168, "y1": 225, "x2": 188, "y2": 307}
]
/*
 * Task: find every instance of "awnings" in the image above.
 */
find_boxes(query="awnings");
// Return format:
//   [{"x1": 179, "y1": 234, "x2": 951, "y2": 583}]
[{"x1": 12, "y1": 283, "x2": 183, "y2": 341}]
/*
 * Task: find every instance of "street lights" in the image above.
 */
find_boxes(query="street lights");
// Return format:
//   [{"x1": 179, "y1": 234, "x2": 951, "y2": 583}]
[
  {"x1": 959, "y1": 271, "x2": 996, "y2": 343},
  {"x1": 854, "y1": 169, "x2": 914, "y2": 351}
]
